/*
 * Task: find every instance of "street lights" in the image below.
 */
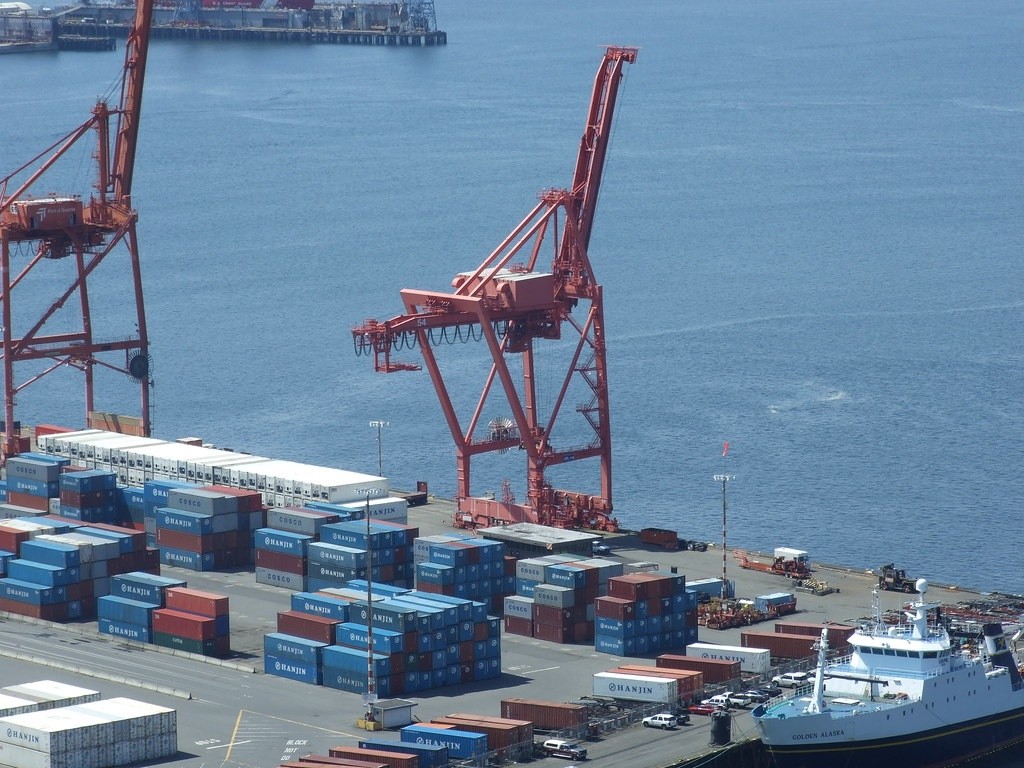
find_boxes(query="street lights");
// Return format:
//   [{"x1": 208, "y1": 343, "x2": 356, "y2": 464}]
[
  {"x1": 368, "y1": 421, "x2": 390, "y2": 478},
  {"x1": 712, "y1": 473, "x2": 736, "y2": 596},
  {"x1": 355, "y1": 485, "x2": 381, "y2": 720}
]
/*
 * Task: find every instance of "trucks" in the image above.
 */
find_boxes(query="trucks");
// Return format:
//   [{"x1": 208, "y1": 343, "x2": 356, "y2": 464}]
[
  {"x1": 592, "y1": 540, "x2": 610, "y2": 556},
  {"x1": 722, "y1": 692, "x2": 752, "y2": 709}
]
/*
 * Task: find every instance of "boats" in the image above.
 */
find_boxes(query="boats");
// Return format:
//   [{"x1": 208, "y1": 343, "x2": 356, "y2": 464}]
[{"x1": 751, "y1": 578, "x2": 1024, "y2": 768}]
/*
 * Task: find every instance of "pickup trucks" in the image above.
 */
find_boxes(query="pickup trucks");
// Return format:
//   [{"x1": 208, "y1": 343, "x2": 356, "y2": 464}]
[
  {"x1": 542, "y1": 739, "x2": 587, "y2": 760},
  {"x1": 660, "y1": 711, "x2": 690, "y2": 725}
]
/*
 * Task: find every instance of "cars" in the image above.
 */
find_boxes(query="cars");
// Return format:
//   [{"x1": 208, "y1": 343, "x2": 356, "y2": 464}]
[
  {"x1": 688, "y1": 704, "x2": 714, "y2": 716},
  {"x1": 736, "y1": 686, "x2": 783, "y2": 703}
]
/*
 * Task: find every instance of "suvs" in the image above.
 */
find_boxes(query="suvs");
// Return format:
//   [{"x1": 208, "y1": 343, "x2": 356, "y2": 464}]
[
  {"x1": 641, "y1": 714, "x2": 677, "y2": 730},
  {"x1": 701, "y1": 695, "x2": 731, "y2": 711},
  {"x1": 772, "y1": 673, "x2": 808, "y2": 689}
]
